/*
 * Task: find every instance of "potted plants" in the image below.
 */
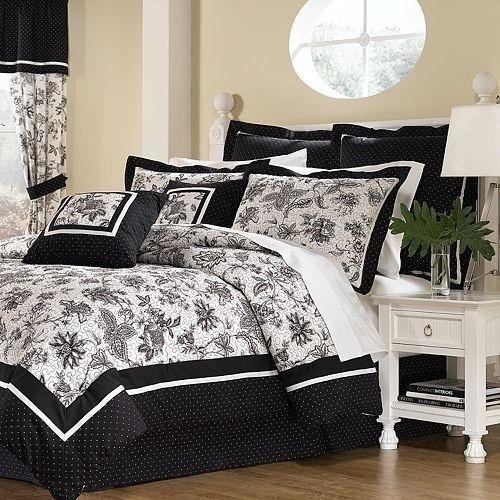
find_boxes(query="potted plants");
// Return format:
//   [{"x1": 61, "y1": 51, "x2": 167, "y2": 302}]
[{"x1": 388, "y1": 198, "x2": 495, "y2": 291}]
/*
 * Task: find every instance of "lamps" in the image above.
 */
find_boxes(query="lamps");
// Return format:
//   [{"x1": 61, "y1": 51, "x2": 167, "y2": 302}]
[{"x1": 441, "y1": 105, "x2": 500, "y2": 294}]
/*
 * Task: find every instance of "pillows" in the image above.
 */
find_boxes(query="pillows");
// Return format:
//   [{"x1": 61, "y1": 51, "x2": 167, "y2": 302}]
[
  {"x1": 125, "y1": 156, "x2": 270, "y2": 191},
  {"x1": 331, "y1": 122, "x2": 478, "y2": 291},
  {"x1": 283, "y1": 160, "x2": 426, "y2": 279},
  {"x1": 170, "y1": 149, "x2": 307, "y2": 168},
  {"x1": 222, "y1": 120, "x2": 332, "y2": 169},
  {"x1": 157, "y1": 179, "x2": 248, "y2": 229},
  {"x1": 20, "y1": 190, "x2": 169, "y2": 268},
  {"x1": 231, "y1": 165, "x2": 412, "y2": 296}
]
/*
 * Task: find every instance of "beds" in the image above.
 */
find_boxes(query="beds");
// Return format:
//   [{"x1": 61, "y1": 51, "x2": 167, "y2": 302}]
[{"x1": 0, "y1": 71, "x2": 499, "y2": 499}]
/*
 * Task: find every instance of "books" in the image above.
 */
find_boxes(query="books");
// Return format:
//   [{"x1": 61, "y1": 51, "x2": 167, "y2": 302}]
[
  {"x1": 397, "y1": 394, "x2": 500, "y2": 411},
  {"x1": 405, "y1": 391, "x2": 500, "y2": 404},
  {"x1": 408, "y1": 377, "x2": 500, "y2": 398}
]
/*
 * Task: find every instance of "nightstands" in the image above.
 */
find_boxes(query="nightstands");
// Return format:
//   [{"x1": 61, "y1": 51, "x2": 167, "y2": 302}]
[{"x1": 377, "y1": 292, "x2": 500, "y2": 464}]
